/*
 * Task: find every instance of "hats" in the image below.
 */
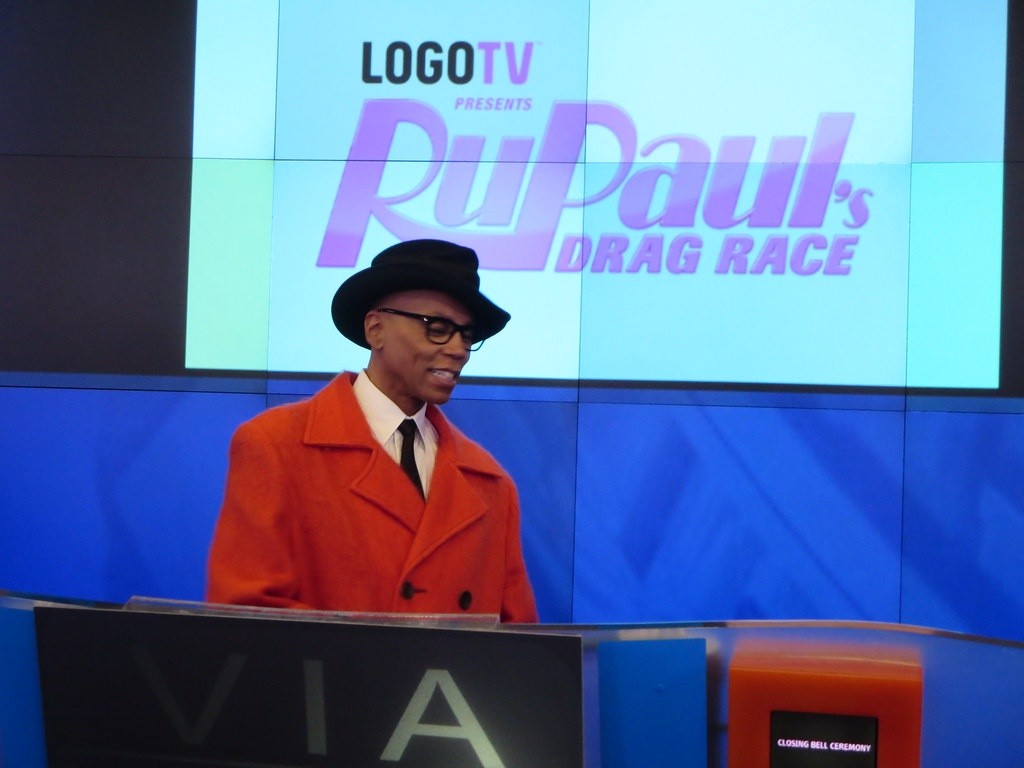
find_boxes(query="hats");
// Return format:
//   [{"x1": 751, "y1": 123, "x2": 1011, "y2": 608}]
[{"x1": 330, "y1": 237, "x2": 511, "y2": 350}]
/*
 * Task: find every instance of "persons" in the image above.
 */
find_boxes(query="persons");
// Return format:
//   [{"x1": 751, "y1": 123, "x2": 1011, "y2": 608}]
[{"x1": 206, "y1": 237, "x2": 540, "y2": 624}]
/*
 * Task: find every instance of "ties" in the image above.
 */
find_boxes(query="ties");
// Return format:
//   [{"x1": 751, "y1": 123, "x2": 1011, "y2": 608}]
[{"x1": 400, "y1": 418, "x2": 426, "y2": 496}]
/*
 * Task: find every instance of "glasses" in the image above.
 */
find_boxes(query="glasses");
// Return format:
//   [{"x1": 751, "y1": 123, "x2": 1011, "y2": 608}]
[{"x1": 377, "y1": 307, "x2": 486, "y2": 351}]
